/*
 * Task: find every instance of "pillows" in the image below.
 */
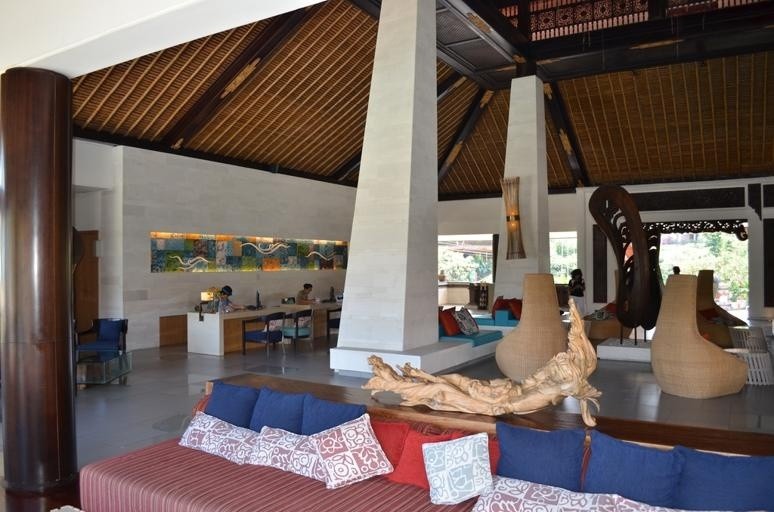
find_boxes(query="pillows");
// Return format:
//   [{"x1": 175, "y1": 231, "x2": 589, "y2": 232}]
[
  {"x1": 452, "y1": 430, "x2": 499, "y2": 475},
  {"x1": 471, "y1": 473, "x2": 676, "y2": 512},
  {"x1": 250, "y1": 387, "x2": 305, "y2": 434},
  {"x1": 582, "y1": 430, "x2": 682, "y2": 510},
  {"x1": 674, "y1": 444, "x2": 773, "y2": 511},
  {"x1": 302, "y1": 393, "x2": 368, "y2": 437},
  {"x1": 421, "y1": 431, "x2": 493, "y2": 506},
  {"x1": 369, "y1": 420, "x2": 410, "y2": 469},
  {"x1": 439, "y1": 306, "x2": 479, "y2": 336},
  {"x1": 249, "y1": 425, "x2": 325, "y2": 483},
  {"x1": 491, "y1": 296, "x2": 522, "y2": 320},
  {"x1": 203, "y1": 383, "x2": 260, "y2": 429},
  {"x1": 309, "y1": 413, "x2": 394, "y2": 490},
  {"x1": 388, "y1": 428, "x2": 463, "y2": 489},
  {"x1": 496, "y1": 420, "x2": 586, "y2": 492}
]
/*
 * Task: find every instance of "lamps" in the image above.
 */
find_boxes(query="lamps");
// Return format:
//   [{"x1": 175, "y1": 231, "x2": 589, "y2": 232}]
[
  {"x1": 499, "y1": 176, "x2": 527, "y2": 260},
  {"x1": 195, "y1": 291, "x2": 214, "y2": 321}
]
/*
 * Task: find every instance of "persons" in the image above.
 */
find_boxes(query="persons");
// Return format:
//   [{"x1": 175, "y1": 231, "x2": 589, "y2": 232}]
[
  {"x1": 204, "y1": 285, "x2": 249, "y2": 314},
  {"x1": 673, "y1": 266, "x2": 680, "y2": 275},
  {"x1": 567, "y1": 269, "x2": 589, "y2": 318},
  {"x1": 295, "y1": 282, "x2": 316, "y2": 306}
]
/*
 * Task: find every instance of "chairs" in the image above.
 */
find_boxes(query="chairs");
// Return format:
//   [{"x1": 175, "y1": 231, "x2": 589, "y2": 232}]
[
  {"x1": 650, "y1": 270, "x2": 748, "y2": 399},
  {"x1": 581, "y1": 270, "x2": 633, "y2": 340},
  {"x1": 75, "y1": 318, "x2": 128, "y2": 362},
  {"x1": 495, "y1": 273, "x2": 567, "y2": 383},
  {"x1": 439, "y1": 325, "x2": 503, "y2": 362}
]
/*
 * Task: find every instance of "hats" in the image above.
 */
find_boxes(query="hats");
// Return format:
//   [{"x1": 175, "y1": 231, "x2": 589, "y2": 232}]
[{"x1": 222, "y1": 286, "x2": 232, "y2": 295}]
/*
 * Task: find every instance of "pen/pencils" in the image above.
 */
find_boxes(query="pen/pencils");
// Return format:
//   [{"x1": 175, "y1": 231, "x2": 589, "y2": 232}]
[{"x1": 273, "y1": 306, "x2": 280, "y2": 307}]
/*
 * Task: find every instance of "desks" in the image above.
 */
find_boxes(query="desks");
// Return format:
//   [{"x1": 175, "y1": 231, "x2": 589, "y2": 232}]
[
  {"x1": 721, "y1": 326, "x2": 774, "y2": 385},
  {"x1": 187, "y1": 299, "x2": 342, "y2": 356},
  {"x1": 75, "y1": 350, "x2": 134, "y2": 390}
]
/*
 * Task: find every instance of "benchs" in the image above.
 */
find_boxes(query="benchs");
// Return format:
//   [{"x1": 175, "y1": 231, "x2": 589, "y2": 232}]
[
  {"x1": 80, "y1": 373, "x2": 774, "y2": 512},
  {"x1": 472, "y1": 309, "x2": 519, "y2": 337}
]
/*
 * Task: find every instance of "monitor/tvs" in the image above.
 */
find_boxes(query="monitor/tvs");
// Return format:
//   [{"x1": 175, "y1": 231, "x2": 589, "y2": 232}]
[
  {"x1": 330, "y1": 286, "x2": 336, "y2": 302},
  {"x1": 255, "y1": 289, "x2": 262, "y2": 309}
]
